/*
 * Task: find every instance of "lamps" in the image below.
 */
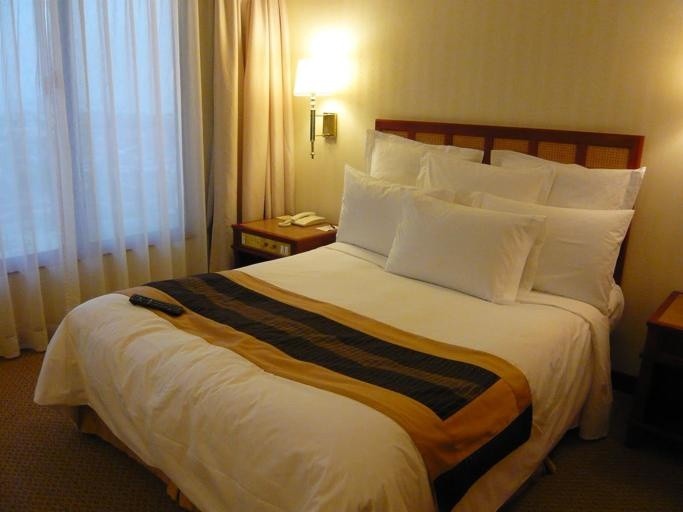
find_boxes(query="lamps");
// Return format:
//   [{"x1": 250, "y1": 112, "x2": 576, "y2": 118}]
[{"x1": 292, "y1": 59, "x2": 336, "y2": 160}]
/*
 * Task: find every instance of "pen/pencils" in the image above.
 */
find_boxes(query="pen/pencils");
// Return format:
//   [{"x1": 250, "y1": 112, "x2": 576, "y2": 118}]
[{"x1": 330, "y1": 223, "x2": 335, "y2": 229}]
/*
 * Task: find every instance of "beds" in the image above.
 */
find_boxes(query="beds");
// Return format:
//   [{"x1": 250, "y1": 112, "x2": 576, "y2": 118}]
[{"x1": 32, "y1": 118, "x2": 647, "y2": 512}]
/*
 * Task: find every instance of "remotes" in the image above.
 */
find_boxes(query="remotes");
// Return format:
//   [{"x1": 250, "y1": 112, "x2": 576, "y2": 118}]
[{"x1": 129, "y1": 293, "x2": 187, "y2": 318}]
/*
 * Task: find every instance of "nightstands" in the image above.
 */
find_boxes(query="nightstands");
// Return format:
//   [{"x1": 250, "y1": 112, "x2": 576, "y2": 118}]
[
  {"x1": 230, "y1": 214, "x2": 337, "y2": 269},
  {"x1": 624, "y1": 290, "x2": 683, "y2": 457}
]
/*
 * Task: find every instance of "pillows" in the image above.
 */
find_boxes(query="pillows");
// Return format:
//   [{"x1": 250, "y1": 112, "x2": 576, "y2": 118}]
[
  {"x1": 335, "y1": 164, "x2": 456, "y2": 260},
  {"x1": 383, "y1": 187, "x2": 548, "y2": 305},
  {"x1": 414, "y1": 148, "x2": 556, "y2": 211},
  {"x1": 466, "y1": 191, "x2": 635, "y2": 318},
  {"x1": 490, "y1": 148, "x2": 647, "y2": 212},
  {"x1": 362, "y1": 128, "x2": 485, "y2": 187}
]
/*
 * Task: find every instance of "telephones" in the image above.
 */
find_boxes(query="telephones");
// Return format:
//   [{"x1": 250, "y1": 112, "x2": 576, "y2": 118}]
[{"x1": 289, "y1": 211, "x2": 326, "y2": 226}]
